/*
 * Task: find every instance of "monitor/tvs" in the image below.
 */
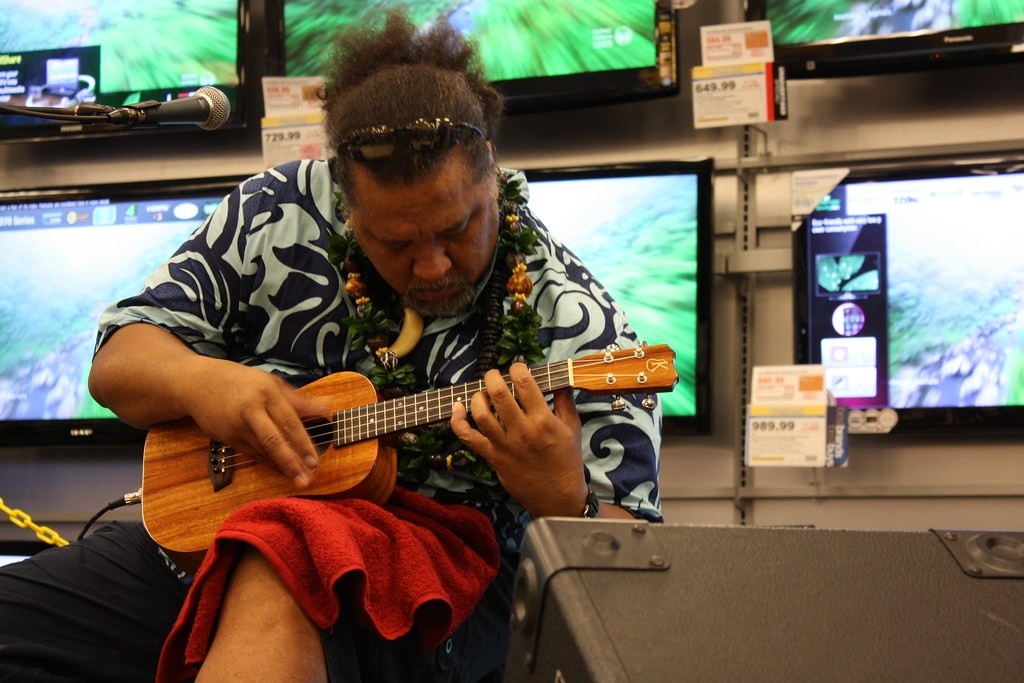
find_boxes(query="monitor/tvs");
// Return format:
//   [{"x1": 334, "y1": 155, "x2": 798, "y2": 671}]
[
  {"x1": 744, "y1": 0, "x2": 1024, "y2": 79},
  {"x1": 791, "y1": 150, "x2": 1024, "y2": 439},
  {"x1": 0, "y1": 0, "x2": 717, "y2": 447}
]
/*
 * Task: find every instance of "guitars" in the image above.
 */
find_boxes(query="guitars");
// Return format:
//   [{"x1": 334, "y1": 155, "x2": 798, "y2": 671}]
[{"x1": 140, "y1": 340, "x2": 681, "y2": 580}]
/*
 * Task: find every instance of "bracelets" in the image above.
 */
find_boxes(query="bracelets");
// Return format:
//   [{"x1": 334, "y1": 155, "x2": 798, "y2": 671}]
[{"x1": 578, "y1": 486, "x2": 600, "y2": 518}]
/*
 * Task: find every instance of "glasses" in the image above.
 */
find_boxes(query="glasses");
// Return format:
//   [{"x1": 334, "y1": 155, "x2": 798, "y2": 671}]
[{"x1": 337, "y1": 118, "x2": 484, "y2": 160}]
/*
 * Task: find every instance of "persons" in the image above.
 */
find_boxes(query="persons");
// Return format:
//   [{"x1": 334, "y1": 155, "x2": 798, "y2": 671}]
[{"x1": 0, "y1": 12, "x2": 663, "y2": 683}]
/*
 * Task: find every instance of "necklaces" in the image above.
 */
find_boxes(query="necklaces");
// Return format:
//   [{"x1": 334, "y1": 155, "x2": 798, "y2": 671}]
[{"x1": 341, "y1": 173, "x2": 546, "y2": 470}]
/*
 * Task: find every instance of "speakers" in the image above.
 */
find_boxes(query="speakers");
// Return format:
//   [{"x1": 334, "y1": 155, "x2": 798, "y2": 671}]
[{"x1": 497, "y1": 517, "x2": 1024, "y2": 683}]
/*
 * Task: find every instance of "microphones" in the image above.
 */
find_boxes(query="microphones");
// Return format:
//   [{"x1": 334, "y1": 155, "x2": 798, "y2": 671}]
[{"x1": 108, "y1": 86, "x2": 232, "y2": 131}]
